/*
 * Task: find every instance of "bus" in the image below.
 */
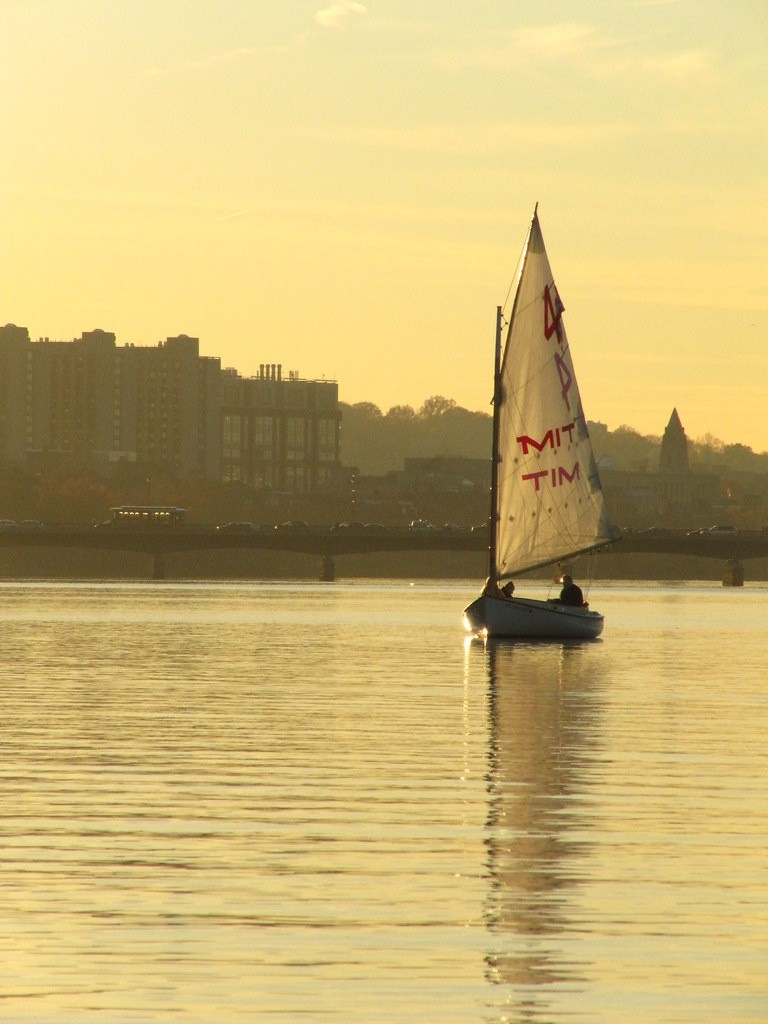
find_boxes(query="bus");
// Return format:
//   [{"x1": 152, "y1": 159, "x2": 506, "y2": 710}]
[{"x1": 110, "y1": 504, "x2": 188, "y2": 527}]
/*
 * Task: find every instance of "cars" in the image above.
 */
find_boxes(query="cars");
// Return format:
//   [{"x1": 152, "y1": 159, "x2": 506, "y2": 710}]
[
  {"x1": 636, "y1": 520, "x2": 738, "y2": 538},
  {"x1": 2, "y1": 518, "x2": 47, "y2": 528},
  {"x1": 212, "y1": 516, "x2": 486, "y2": 532}
]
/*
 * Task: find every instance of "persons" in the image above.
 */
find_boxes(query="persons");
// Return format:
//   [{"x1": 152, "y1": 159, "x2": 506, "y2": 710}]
[
  {"x1": 547, "y1": 575, "x2": 583, "y2": 606},
  {"x1": 501, "y1": 581, "x2": 515, "y2": 598}
]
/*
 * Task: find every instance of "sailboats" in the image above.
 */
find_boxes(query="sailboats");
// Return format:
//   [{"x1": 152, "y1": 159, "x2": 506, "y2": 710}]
[{"x1": 461, "y1": 200, "x2": 618, "y2": 641}]
